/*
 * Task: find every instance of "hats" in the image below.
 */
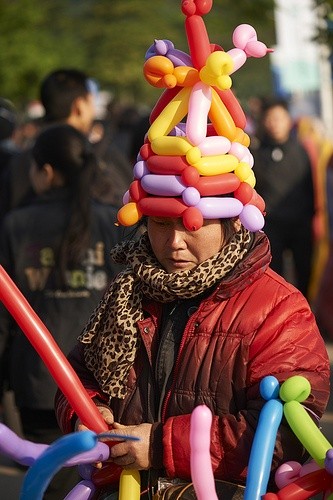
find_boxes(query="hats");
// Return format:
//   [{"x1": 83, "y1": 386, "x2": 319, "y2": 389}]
[{"x1": 113, "y1": 0, "x2": 268, "y2": 235}]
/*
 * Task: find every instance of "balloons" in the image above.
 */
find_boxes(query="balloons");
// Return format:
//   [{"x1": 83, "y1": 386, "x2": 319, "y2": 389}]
[
  {"x1": 243, "y1": 375, "x2": 333, "y2": 500},
  {"x1": 115, "y1": 0, "x2": 275, "y2": 233},
  {"x1": 189, "y1": 405, "x2": 219, "y2": 500},
  {"x1": 0, "y1": 264, "x2": 142, "y2": 500}
]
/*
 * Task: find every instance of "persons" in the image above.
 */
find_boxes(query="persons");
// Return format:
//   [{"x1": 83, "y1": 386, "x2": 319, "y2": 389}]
[
  {"x1": 249, "y1": 101, "x2": 316, "y2": 294},
  {"x1": 55, "y1": 193, "x2": 331, "y2": 500},
  {"x1": 0, "y1": 124, "x2": 130, "y2": 500},
  {"x1": 0, "y1": 67, "x2": 152, "y2": 222}
]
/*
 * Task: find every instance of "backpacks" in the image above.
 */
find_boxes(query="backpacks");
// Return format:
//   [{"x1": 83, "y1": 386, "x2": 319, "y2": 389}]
[{"x1": 153, "y1": 479, "x2": 248, "y2": 500}]
